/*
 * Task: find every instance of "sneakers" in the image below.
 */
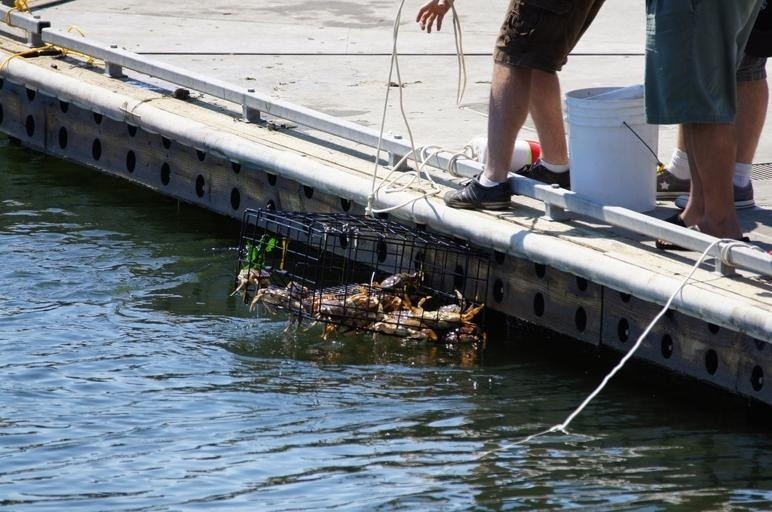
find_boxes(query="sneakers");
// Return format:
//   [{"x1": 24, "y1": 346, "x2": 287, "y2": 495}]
[
  {"x1": 443, "y1": 168, "x2": 515, "y2": 211},
  {"x1": 731, "y1": 179, "x2": 755, "y2": 211},
  {"x1": 654, "y1": 163, "x2": 692, "y2": 201},
  {"x1": 514, "y1": 159, "x2": 571, "y2": 190}
]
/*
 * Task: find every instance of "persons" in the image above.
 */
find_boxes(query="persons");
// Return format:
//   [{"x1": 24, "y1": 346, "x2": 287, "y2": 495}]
[
  {"x1": 414, "y1": 0, "x2": 606, "y2": 210},
  {"x1": 654, "y1": 0, "x2": 772, "y2": 214},
  {"x1": 642, "y1": 1, "x2": 761, "y2": 253}
]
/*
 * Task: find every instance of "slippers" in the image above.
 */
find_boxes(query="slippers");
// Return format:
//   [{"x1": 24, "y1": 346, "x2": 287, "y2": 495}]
[
  {"x1": 654, "y1": 226, "x2": 751, "y2": 254},
  {"x1": 665, "y1": 213, "x2": 689, "y2": 228}
]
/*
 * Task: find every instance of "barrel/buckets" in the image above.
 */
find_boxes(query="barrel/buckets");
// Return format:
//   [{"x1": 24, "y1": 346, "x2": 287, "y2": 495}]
[{"x1": 565, "y1": 87, "x2": 665, "y2": 213}]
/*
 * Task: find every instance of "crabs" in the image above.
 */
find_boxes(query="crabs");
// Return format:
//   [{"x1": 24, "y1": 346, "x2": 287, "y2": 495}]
[{"x1": 227, "y1": 263, "x2": 489, "y2": 349}]
